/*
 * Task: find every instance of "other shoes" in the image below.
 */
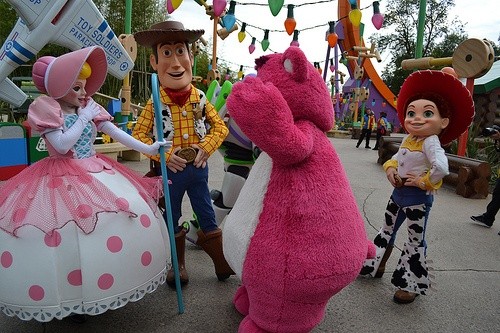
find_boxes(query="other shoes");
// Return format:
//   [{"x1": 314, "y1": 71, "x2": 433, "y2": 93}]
[
  {"x1": 365, "y1": 145, "x2": 371, "y2": 148},
  {"x1": 471, "y1": 215, "x2": 495, "y2": 228},
  {"x1": 373, "y1": 147, "x2": 379, "y2": 150},
  {"x1": 356, "y1": 144, "x2": 359, "y2": 148}
]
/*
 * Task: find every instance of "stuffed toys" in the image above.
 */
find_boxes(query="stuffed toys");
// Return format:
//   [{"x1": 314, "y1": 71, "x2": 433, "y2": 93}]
[{"x1": 221, "y1": 46, "x2": 378, "y2": 333}]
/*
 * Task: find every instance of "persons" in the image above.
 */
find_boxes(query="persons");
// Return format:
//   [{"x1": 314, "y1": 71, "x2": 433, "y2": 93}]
[
  {"x1": 358, "y1": 66, "x2": 477, "y2": 305},
  {"x1": 127, "y1": 19, "x2": 238, "y2": 290},
  {"x1": 470, "y1": 175, "x2": 500, "y2": 237},
  {"x1": 355, "y1": 107, "x2": 372, "y2": 149},
  {"x1": 0, "y1": 45, "x2": 176, "y2": 324},
  {"x1": 182, "y1": 75, "x2": 262, "y2": 246},
  {"x1": 372, "y1": 111, "x2": 392, "y2": 150}
]
